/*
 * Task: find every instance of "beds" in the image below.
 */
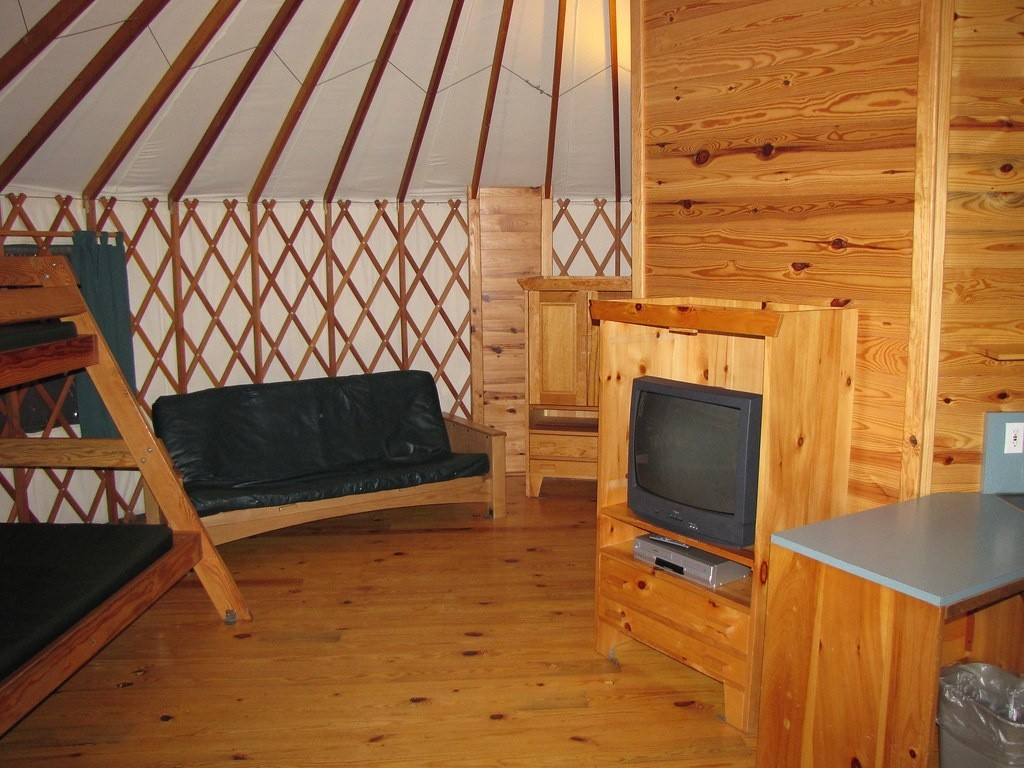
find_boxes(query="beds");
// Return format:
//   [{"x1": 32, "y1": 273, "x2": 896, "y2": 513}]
[{"x1": 0, "y1": 254, "x2": 255, "y2": 741}]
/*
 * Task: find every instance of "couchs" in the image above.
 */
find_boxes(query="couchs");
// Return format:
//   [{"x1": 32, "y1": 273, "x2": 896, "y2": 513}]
[{"x1": 152, "y1": 369, "x2": 507, "y2": 546}]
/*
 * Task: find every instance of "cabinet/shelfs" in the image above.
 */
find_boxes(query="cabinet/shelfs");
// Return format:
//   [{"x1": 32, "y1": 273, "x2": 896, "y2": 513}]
[
  {"x1": 516, "y1": 274, "x2": 633, "y2": 499},
  {"x1": 586, "y1": 294, "x2": 862, "y2": 740}
]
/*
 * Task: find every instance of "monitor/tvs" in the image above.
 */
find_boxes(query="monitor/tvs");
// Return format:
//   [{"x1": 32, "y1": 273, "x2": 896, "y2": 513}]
[{"x1": 626, "y1": 374, "x2": 762, "y2": 550}]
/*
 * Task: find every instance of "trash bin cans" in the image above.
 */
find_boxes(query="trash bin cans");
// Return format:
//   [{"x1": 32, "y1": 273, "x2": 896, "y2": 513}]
[{"x1": 938, "y1": 661, "x2": 1024, "y2": 768}]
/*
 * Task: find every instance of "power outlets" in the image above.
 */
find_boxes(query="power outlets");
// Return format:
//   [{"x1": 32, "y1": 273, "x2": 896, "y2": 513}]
[{"x1": 1004, "y1": 422, "x2": 1024, "y2": 453}]
